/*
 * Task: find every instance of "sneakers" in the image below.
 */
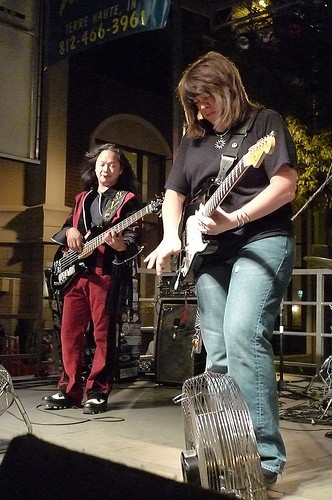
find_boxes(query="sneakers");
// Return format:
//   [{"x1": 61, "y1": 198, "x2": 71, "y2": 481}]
[
  {"x1": 42, "y1": 392, "x2": 79, "y2": 408},
  {"x1": 82, "y1": 397, "x2": 107, "y2": 413}
]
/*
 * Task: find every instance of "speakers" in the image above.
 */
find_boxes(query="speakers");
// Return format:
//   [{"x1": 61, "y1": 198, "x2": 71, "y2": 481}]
[
  {"x1": 154, "y1": 302, "x2": 207, "y2": 387},
  {"x1": 0, "y1": 433, "x2": 243, "y2": 500}
]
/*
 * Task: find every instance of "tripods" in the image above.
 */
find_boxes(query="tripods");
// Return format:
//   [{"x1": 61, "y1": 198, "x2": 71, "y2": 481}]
[{"x1": 277, "y1": 303, "x2": 320, "y2": 401}]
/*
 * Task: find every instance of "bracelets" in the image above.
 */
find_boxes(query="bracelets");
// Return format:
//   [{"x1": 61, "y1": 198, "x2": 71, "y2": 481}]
[{"x1": 235, "y1": 208, "x2": 250, "y2": 227}]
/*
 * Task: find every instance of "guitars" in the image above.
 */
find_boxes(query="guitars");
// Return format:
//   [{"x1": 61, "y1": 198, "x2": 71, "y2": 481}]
[
  {"x1": 178, "y1": 129, "x2": 276, "y2": 283},
  {"x1": 51, "y1": 191, "x2": 165, "y2": 292}
]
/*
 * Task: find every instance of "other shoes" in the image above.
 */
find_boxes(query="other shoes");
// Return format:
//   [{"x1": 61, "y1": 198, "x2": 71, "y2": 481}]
[{"x1": 260, "y1": 468, "x2": 278, "y2": 489}]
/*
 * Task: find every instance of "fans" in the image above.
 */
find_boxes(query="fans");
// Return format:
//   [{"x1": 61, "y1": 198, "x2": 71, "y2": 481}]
[
  {"x1": 172, "y1": 371, "x2": 268, "y2": 500},
  {"x1": 0, "y1": 364, "x2": 32, "y2": 454}
]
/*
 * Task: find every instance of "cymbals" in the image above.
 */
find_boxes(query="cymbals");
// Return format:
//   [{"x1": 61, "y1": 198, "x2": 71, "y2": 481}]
[{"x1": 303, "y1": 256, "x2": 332, "y2": 268}]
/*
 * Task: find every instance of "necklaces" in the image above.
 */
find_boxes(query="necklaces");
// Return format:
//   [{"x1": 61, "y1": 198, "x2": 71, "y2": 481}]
[{"x1": 215, "y1": 128, "x2": 229, "y2": 149}]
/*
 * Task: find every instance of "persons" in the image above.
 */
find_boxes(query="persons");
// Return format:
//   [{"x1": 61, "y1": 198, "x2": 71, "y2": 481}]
[
  {"x1": 143, "y1": 51, "x2": 297, "y2": 488},
  {"x1": 42, "y1": 143, "x2": 143, "y2": 414}
]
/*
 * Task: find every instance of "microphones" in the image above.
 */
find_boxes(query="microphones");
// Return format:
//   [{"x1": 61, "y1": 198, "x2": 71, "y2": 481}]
[{"x1": 172, "y1": 318, "x2": 182, "y2": 339}]
